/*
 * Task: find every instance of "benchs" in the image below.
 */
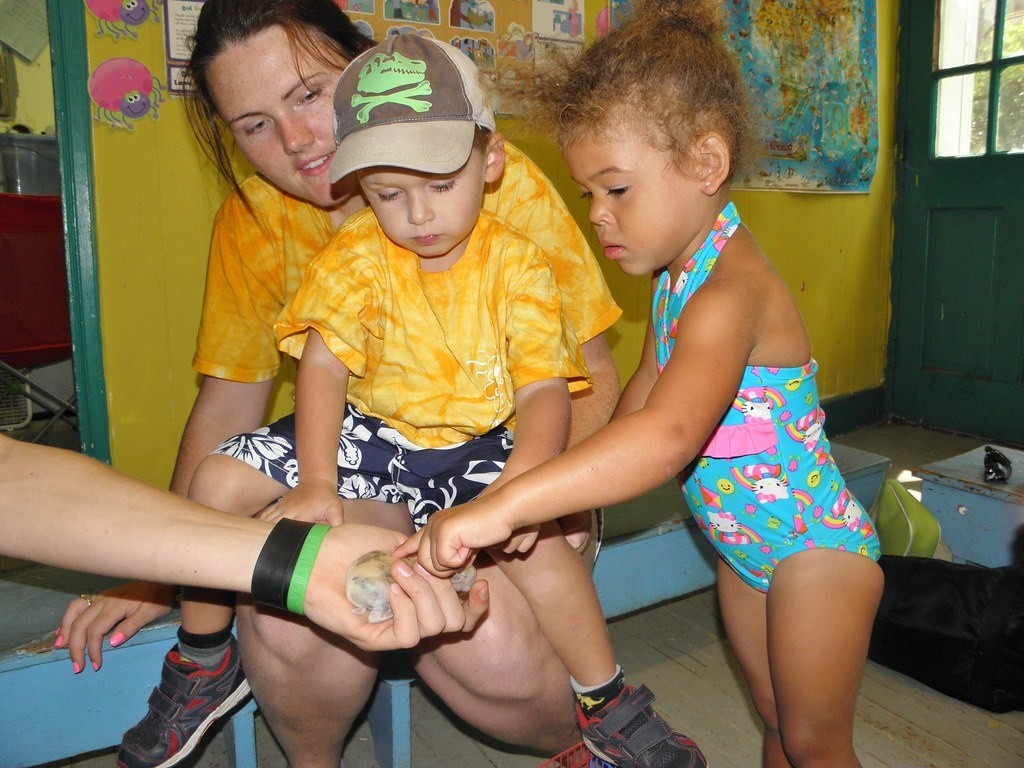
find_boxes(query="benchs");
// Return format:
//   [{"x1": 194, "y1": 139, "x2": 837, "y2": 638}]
[{"x1": 0, "y1": 439, "x2": 889, "y2": 768}]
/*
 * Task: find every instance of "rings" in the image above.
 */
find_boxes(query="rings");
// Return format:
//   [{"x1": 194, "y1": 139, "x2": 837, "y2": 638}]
[{"x1": 79, "y1": 591, "x2": 94, "y2": 605}]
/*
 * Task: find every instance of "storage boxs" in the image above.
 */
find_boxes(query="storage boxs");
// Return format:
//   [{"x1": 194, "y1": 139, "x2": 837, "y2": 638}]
[{"x1": 0, "y1": 133, "x2": 61, "y2": 195}]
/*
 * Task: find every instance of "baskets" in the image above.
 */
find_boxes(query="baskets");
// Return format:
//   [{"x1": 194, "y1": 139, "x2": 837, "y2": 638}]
[{"x1": 536, "y1": 742, "x2": 612, "y2": 768}]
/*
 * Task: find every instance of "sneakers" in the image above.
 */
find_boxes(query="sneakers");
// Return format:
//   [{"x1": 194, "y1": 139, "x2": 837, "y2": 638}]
[
  {"x1": 117, "y1": 633, "x2": 254, "y2": 768},
  {"x1": 578, "y1": 671, "x2": 709, "y2": 767}
]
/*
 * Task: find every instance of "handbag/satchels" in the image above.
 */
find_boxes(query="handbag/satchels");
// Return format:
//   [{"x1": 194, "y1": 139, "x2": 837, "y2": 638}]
[
  {"x1": 868, "y1": 523, "x2": 1024, "y2": 713},
  {"x1": 875, "y1": 479, "x2": 940, "y2": 559}
]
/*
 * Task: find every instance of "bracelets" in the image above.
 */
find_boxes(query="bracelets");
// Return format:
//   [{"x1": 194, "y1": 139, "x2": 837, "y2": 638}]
[{"x1": 251, "y1": 517, "x2": 333, "y2": 620}]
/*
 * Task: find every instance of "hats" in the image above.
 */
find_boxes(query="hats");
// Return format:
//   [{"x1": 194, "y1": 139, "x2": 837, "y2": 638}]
[{"x1": 329, "y1": 32, "x2": 496, "y2": 183}]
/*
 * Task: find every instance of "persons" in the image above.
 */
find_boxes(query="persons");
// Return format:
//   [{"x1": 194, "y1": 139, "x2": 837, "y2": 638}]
[
  {"x1": 115, "y1": 33, "x2": 709, "y2": 768},
  {"x1": 53, "y1": 0, "x2": 625, "y2": 768},
  {"x1": 393, "y1": 0, "x2": 886, "y2": 768},
  {"x1": 0, "y1": 431, "x2": 491, "y2": 653}
]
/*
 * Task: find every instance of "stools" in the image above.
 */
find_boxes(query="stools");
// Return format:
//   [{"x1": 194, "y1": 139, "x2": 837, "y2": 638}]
[{"x1": 913, "y1": 444, "x2": 1024, "y2": 568}]
